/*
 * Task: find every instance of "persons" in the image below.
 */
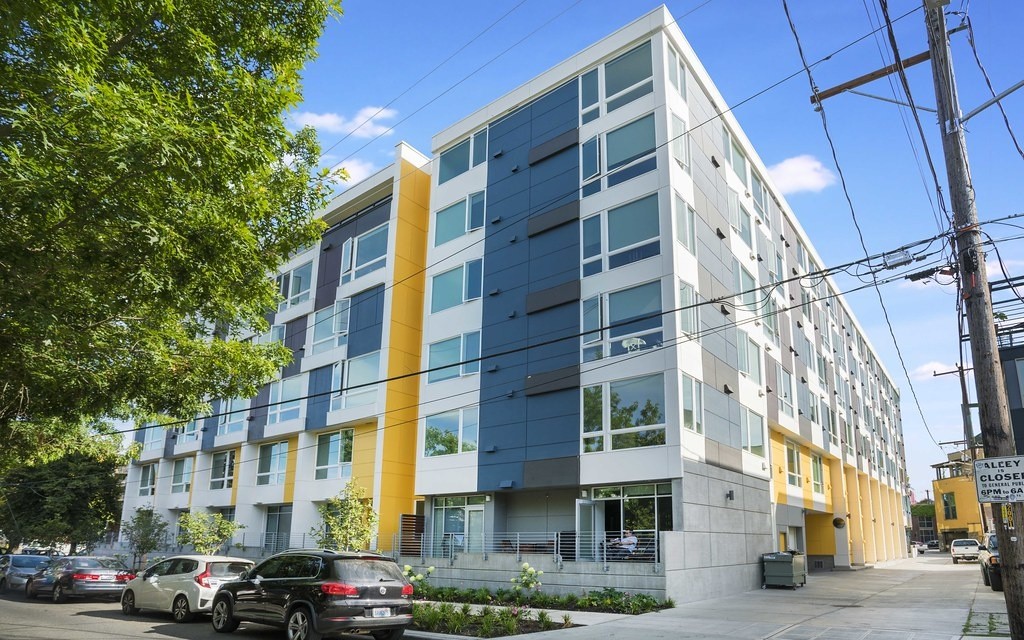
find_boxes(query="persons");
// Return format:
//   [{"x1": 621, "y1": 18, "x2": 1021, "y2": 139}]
[{"x1": 608, "y1": 528, "x2": 637, "y2": 561}]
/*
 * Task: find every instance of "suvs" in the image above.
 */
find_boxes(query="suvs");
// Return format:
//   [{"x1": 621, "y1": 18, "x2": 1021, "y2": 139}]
[{"x1": 977, "y1": 533, "x2": 1003, "y2": 592}]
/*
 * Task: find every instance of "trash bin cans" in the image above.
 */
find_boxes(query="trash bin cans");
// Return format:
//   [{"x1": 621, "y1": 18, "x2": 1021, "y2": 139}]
[
  {"x1": 761, "y1": 551, "x2": 805, "y2": 591},
  {"x1": 910, "y1": 545, "x2": 917, "y2": 558}
]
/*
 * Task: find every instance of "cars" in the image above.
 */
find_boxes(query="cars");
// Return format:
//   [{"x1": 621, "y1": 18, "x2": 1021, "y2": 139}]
[
  {"x1": 120, "y1": 554, "x2": 256, "y2": 624},
  {"x1": 24, "y1": 555, "x2": 137, "y2": 604},
  {"x1": 209, "y1": 547, "x2": 416, "y2": 640},
  {"x1": 0, "y1": 554, "x2": 52, "y2": 595},
  {"x1": 951, "y1": 538, "x2": 983, "y2": 564},
  {"x1": 910, "y1": 539, "x2": 939, "y2": 554}
]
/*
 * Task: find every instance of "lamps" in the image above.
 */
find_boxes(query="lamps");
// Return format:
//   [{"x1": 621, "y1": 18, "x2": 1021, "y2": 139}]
[
  {"x1": 169, "y1": 434, "x2": 176, "y2": 440},
  {"x1": 201, "y1": 427, "x2": 208, "y2": 431},
  {"x1": 245, "y1": 415, "x2": 254, "y2": 421},
  {"x1": 725, "y1": 490, "x2": 735, "y2": 501}
]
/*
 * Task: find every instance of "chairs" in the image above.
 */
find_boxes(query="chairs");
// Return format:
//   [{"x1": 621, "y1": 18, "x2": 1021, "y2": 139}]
[
  {"x1": 181, "y1": 562, "x2": 194, "y2": 574},
  {"x1": 515, "y1": 542, "x2": 541, "y2": 553},
  {"x1": 544, "y1": 540, "x2": 555, "y2": 553},
  {"x1": 500, "y1": 539, "x2": 517, "y2": 553},
  {"x1": 600, "y1": 535, "x2": 662, "y2": 562}
]
